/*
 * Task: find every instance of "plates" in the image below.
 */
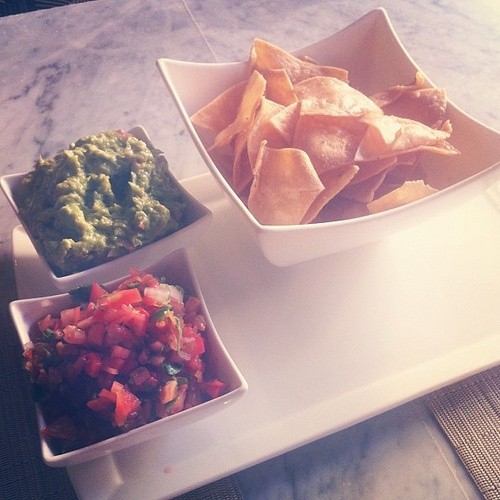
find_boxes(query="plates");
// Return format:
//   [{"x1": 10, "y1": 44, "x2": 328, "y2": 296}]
[{"x1": 10, "y1": 161, "x2": 500, "y2": 499}]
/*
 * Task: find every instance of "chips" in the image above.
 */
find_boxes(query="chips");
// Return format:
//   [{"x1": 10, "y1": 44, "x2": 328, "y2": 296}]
[{"x1": 191, "y1": 37, "x2": 463, "y2": 224}]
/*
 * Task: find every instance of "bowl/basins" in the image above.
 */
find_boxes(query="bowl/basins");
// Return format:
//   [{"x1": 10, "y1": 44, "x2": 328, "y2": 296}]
[
  {"x1": 1, "y1": 125, "x2": 214, "y2": 291},
  {"x1": 9, "y1": 247, "x2": 248, "y2": 467},
  {"x1": 156, "y1": 7, "x2": 499, "y2": 267}
]
